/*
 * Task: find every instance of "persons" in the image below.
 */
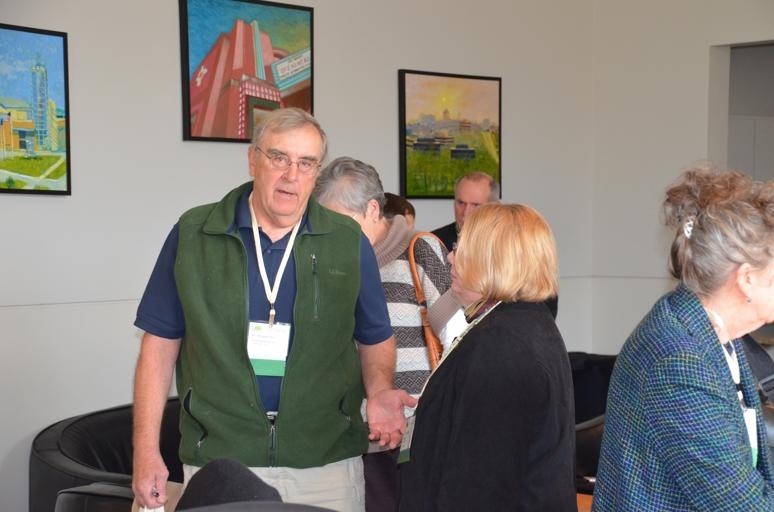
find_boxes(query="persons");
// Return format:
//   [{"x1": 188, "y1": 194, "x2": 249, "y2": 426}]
[
  {"x1": 430, "y1": 171, "x2": 502, "y2": 256},
  {"x1": 382, "y1": 192, "x2": 415, "y2": 228},
  {"x1": 130, "y1": 108, "x2": 418, "y2": 511},
  {"x1": 311, "y1": 157, "x2": 470, "y2": 511},
  {"x1": 590, "y1": 169, "x2": 773, "y2": 511},
  {"x1": 401, "y1": 204, "x2": 578, "y2": 511}
]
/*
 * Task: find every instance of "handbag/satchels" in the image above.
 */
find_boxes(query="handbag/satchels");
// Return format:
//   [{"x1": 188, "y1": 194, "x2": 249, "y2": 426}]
[{"x1": 407, "y1": 230, "x2": 444, "y2": 371}]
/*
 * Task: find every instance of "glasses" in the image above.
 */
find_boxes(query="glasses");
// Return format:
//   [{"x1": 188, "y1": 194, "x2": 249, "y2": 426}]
[{"x1": 255, "y1": 146, "x2": 322, "y2": 173}]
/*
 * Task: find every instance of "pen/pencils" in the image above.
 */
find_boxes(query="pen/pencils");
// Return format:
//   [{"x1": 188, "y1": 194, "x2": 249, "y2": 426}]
[{"x1": 152, "y1": 487, "x2": 160, "y2": 497}]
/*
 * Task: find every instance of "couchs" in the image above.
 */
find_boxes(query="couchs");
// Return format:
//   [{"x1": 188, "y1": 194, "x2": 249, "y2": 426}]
[
  {"x1": 30, "y1": 396, "x2": 180, "y2": 511},
  {"x1": 567, "y1": 351, "x2": 615, "y2": 493}
]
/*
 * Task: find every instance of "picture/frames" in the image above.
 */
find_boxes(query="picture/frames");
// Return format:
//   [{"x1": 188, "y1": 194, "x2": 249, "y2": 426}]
[
  {"x1": 396, "y1": 65, "x2": 504, "y2": 202},
  {"x1": 177, "y1": 0, "x2": 317, "y2": 146},
  {"x1": 0, "y1": 23, "x2": 73, "y2": 197}
]
